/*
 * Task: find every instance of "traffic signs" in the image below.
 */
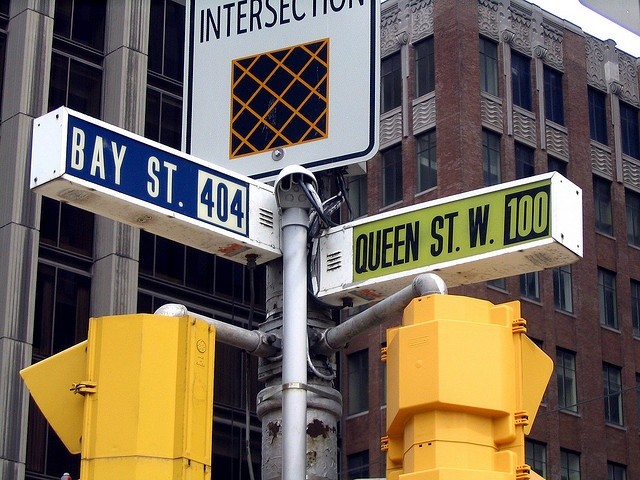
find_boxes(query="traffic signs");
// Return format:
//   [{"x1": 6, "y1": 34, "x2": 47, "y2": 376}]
[{"x1": 181, "y1": 1, "x2": 382, "y2": 182}]
